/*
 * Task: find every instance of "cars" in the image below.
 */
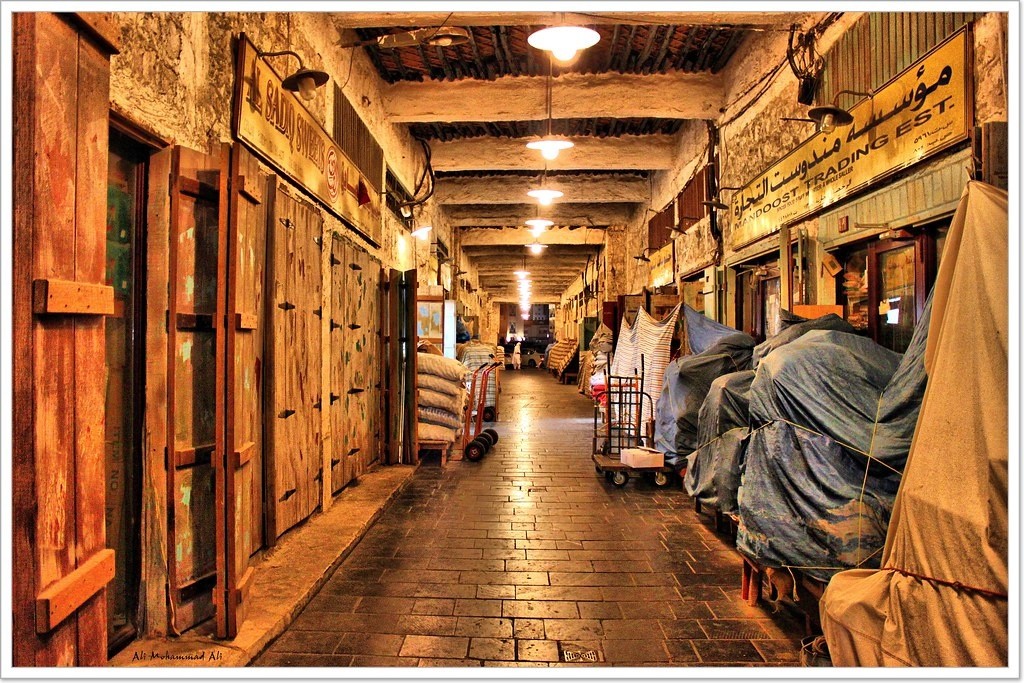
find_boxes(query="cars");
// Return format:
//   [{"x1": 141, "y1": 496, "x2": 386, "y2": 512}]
[
  {"x1": 521, "y1": 348, "x2": 546, "y2": 370},
  {"x1": 504, "y1": 352, "x2": 513, "y2": 367}
]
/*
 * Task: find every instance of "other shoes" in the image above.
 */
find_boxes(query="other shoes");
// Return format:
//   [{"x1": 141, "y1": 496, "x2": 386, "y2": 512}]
[
  {"x1": 518, "y1": 368, "x2": 521, "y2": 370},
  {"x1": 514, "y1": 368, "x2": 516, "y2": 370}
]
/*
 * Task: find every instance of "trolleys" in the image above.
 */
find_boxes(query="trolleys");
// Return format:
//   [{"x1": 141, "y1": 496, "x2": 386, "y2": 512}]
[
  {"x1": 587, "y1": 351, "x2": 669, "y2": 488},
  {"x1": 464, "y1": 351, "x2": 503, "y2": 459}
]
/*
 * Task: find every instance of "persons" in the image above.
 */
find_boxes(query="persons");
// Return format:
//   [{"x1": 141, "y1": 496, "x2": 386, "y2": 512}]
[
  {"x1": 512, "y1": 342, "x2": 522, "y2": 370},
  {"x1": 535, "y1": 355, "x2": 546, "y2": 368}
]
